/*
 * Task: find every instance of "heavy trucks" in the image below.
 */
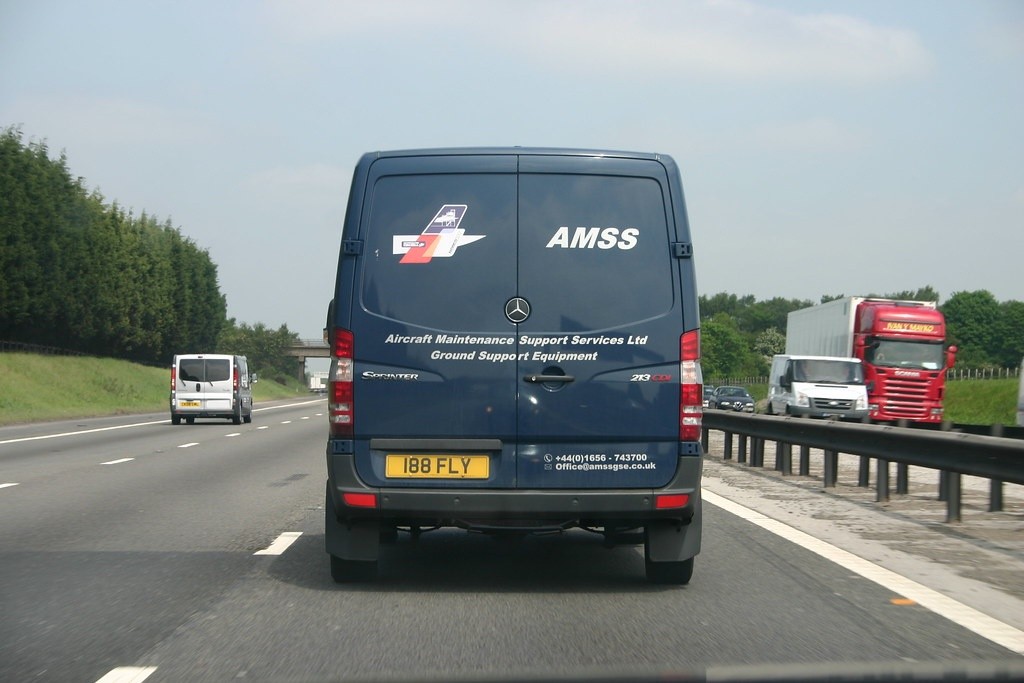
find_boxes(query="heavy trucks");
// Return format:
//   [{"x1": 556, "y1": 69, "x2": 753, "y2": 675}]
[{"x1": 782, "y1": 296, "x2": 958, "y2": 432}]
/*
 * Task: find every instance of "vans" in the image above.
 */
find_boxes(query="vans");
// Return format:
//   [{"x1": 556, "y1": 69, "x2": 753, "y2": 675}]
[
  {"x1": 315, "y1": 149, "x2": 708, "y2": 587},
  {"x1": 171, "y1": 352, "x2": 258, "y2": 426}
]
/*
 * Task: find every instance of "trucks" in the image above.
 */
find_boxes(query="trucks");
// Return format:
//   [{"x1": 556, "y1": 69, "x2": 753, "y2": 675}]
[{"x1": 767, "y1": 351, "x2": 872, "y2": 429}]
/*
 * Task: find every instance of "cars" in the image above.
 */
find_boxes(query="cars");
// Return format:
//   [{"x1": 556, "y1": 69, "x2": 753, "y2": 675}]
[
  {"x1": 709, "y1": 385, "x2": 755, "y2": 415},
  {"x1": 701, "y1": 381, "x2": 716, "y2": 409}
]
